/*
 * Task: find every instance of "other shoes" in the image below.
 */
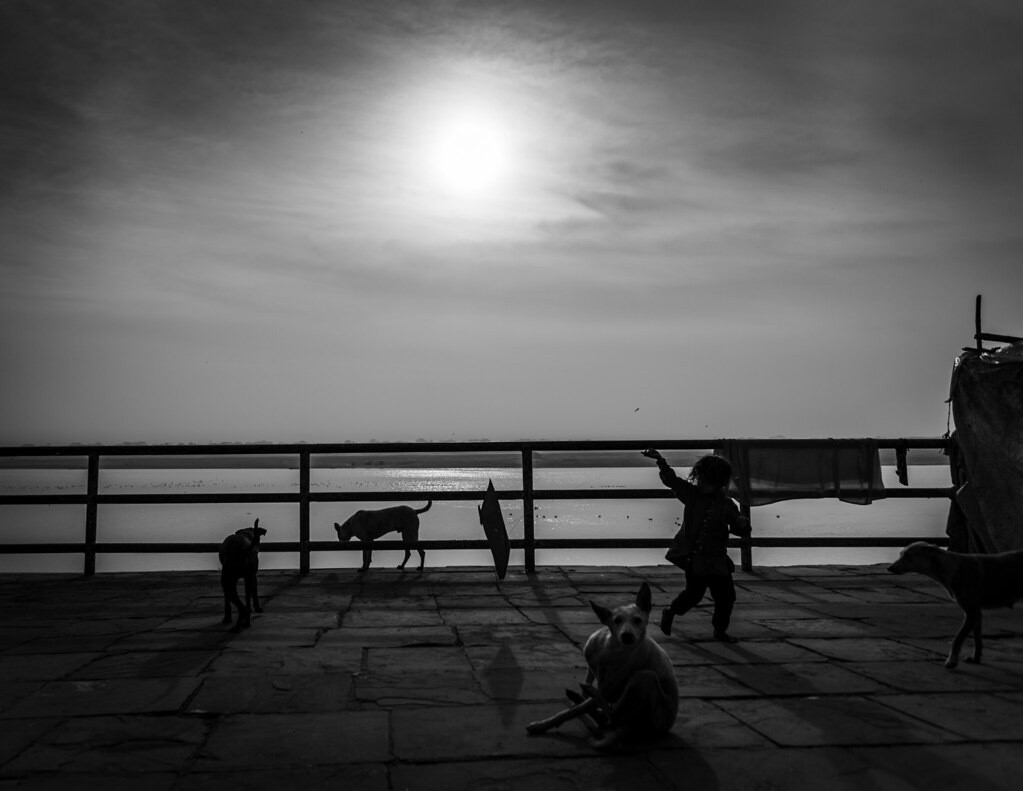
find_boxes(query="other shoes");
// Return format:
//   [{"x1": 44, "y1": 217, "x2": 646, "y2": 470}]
[
  {"x1": 713, "y1": 629, "x2": 738, "y2": 644},
  {"x1": 660, "y1": 607, "x2": 673, "y2": 637}
]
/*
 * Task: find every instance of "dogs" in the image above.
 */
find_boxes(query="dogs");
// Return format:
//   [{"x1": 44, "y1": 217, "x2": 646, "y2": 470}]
[
  {"x1": 218, "y1": 516, "x2": 267, "y2": 634},
  {"x1": 333, "y1": 495, "x2": 433, "y2": 572},
  {"x1": 888, "y1": 542, "x2": 1022, "y2": 669},
  {"x1": 526, "y1": 581, "x2": 681, "y2": 752}
]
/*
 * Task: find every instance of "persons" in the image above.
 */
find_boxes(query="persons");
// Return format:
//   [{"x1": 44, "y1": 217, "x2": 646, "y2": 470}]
[{"x1": 643, "y1": 444, "x2": 752, "y2": 644}]
